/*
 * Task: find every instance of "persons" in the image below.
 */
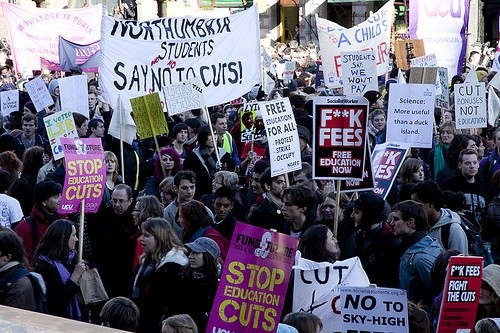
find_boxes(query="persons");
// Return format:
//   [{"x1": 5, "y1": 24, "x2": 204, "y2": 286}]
[
  {"x1": 0, "y1": 35, "x2": 139, "y2": 324},
  {"x1": 99, "y1": 40, "x2": 500, "y2": 333},
  {"x1": 292, "y1": 23, "x2": 300, "y2": 47}
]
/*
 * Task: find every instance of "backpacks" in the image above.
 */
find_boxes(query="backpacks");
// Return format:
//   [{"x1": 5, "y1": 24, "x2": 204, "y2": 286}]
[
  {"x1": 441, "y1": 212, "x2": 484, "y2": 257},
  {"x1": 1, "y1": 269, "x2": 48, "y2": 314}
]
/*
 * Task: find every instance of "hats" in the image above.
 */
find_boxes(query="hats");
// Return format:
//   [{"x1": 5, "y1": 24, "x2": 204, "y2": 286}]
[
  {"x1": 348, "y1": 192, "x2": 382, "y2": 209},
  {"x1": 183, "y1": 237, "x2": 220, "y2": 260},
  {"x1": 297, "y1": 126, "x2": 310, "y2": 141},
  {"x1": 481, "y1": 264, "x2": 500, "y2": 298},
  {"x1": 34, "y1": 178, "x2": 62, "y2": 202}
]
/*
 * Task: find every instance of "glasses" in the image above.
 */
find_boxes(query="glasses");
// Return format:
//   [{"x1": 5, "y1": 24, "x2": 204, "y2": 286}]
[
  {"x1": 113, "y1": 198, "x2": 125, "y2": 204},
  {"x1": 212, "y1": 180, "x2": 223, "y2": 185},
  {"x1": 216, "y1": 202, "x2": 230, "y2": 209}
]
